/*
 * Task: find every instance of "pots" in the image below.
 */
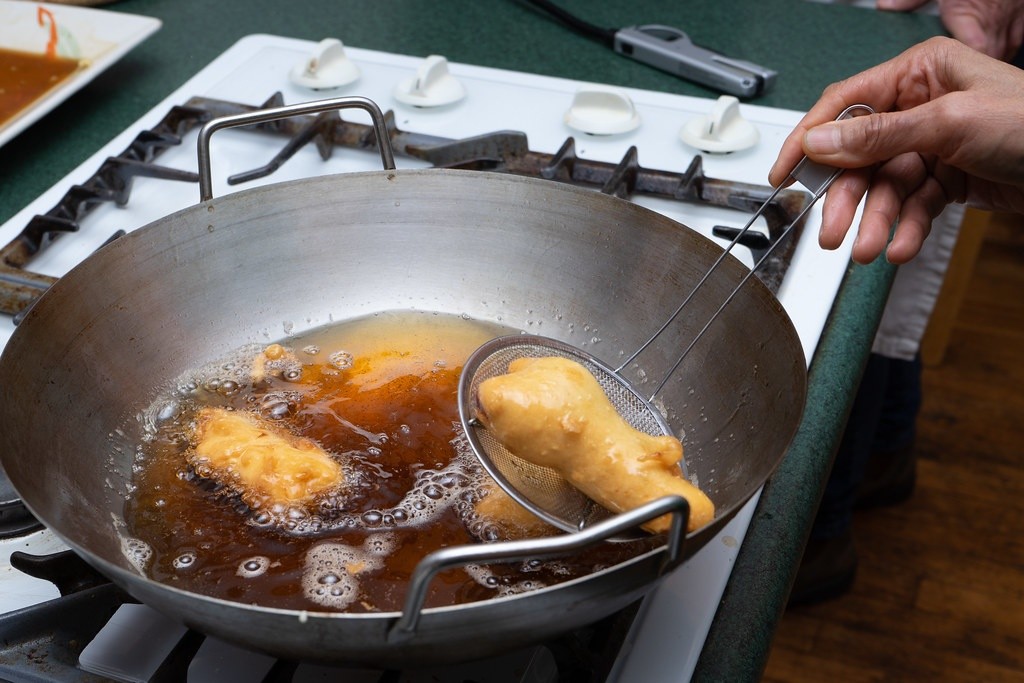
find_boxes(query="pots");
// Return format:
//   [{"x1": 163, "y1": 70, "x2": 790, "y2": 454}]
[{"x1": 0, "y1": 96, "x2": 809, "y2": 664}]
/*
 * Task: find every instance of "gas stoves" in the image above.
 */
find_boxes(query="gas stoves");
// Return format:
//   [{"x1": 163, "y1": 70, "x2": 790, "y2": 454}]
[{"x1": 0, "y1": 33, "x2": 868, "y2": 683}]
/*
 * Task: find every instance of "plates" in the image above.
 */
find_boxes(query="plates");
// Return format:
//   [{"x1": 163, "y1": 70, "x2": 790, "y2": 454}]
[{"x1": 0, "y1": 0, "x2": 164, "y2": 146}]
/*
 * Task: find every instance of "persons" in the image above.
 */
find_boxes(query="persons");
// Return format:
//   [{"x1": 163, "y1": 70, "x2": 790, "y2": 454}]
[{"x1": 770, "y1": 35, "x2": 1024, "y2": 266}]
